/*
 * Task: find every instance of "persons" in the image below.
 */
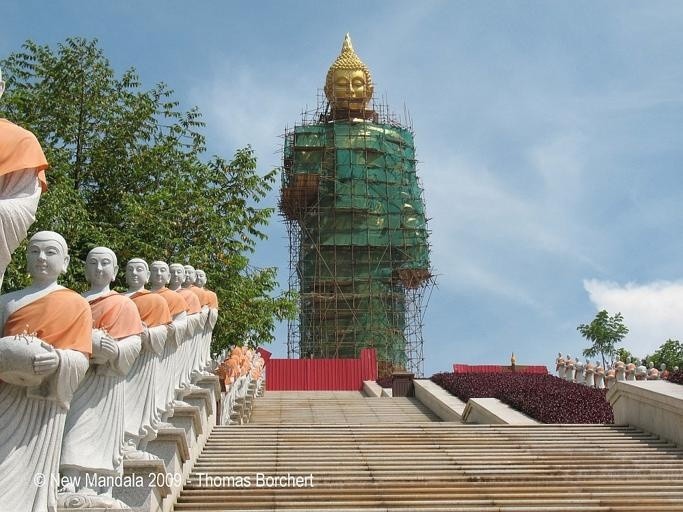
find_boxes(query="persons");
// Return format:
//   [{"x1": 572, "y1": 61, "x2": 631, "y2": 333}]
[
  {"x1": 321, "y1": 33, "x2": 374, "y2": 116},
  {"x1": 555, "y1": 350, "x2": 681, "y2": 394},
  {"x1": 0, "y1": 231, "x2": 265, "y2": 511},
  {"x1": 0, "y1": 68, "x2": 49, "y2": 306}
]
